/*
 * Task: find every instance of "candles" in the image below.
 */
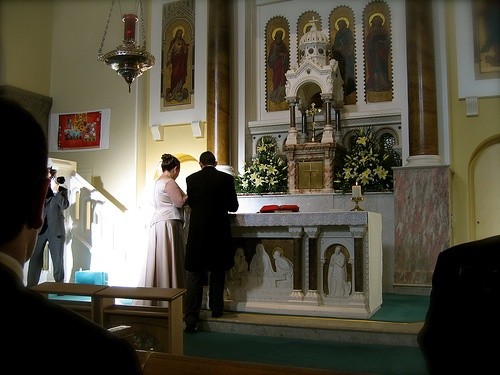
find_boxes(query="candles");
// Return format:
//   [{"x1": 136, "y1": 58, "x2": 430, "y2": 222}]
[{"x1": 353, "y1": 182, "x2": 361, "y2": 197}]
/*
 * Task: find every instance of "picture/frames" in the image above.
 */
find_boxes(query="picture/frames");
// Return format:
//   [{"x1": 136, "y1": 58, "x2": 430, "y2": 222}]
[{"x1": 455, "y1": 0, "x2": 500, "y2": 99}]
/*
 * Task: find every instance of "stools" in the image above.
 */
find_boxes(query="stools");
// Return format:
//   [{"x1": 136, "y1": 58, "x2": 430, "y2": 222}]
[{"x1": 30, "y1": 282, "x2": 187, "y2": 354}]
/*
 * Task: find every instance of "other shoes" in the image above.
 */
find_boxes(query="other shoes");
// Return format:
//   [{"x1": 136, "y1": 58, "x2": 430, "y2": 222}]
[
  {"x1": 210, "y1": 310, "x2": 223, "y2": 318},
  {"x1": 184, "y1": 326, "x2": 196, "y2": 333}
]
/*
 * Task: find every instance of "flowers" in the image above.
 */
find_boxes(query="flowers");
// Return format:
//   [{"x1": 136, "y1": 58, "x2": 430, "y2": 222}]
[
  {"x1": 333, "y1": 126, "x2": 402, "y2": 191},
  {"x1": 235, "y1": 135, "x2": 288, "y2": 195}
]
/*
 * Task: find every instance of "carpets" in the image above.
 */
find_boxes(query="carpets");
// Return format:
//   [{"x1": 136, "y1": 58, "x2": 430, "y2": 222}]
[
  {"x1": 369, "y1": 293, "x2": 430, "y2": 323},
  {"x1": 183, "y1": 329, "x2": 428, "y2": 375}
]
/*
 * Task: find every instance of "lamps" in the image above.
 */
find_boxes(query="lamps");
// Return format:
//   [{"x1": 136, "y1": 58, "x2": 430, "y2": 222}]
[{"x1": 97, "y1": 0, "x2": 155, "y2": 95}]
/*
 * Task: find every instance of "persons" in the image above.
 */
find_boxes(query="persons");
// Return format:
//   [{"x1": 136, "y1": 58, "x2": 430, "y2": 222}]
[
  {"x1": 416, "y1": 234, "x2": 500, "y2": 375},
  {"x1": 0, "y1": 98, "x2": 145, "y2": 375},
  {"x1": 131, "y1": 153, "x2": 188, "y2": 307},
  {"x1": 327, "y1": 246, "x2": 347, "y2": 297},
  {"x1": 233, "y1": 244, "x2": 293, "y2": 281},
  {"x1": 183, "y1": 151, "x2": 239, "y2": 333},
  {"x1": 25, "y1": 177, "x2": 69, "y2": 296}
]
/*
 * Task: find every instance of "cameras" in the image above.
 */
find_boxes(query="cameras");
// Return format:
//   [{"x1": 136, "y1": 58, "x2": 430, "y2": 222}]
[{"x1": 50, "y1": 175, "x2": 65, "y2": 184}]
